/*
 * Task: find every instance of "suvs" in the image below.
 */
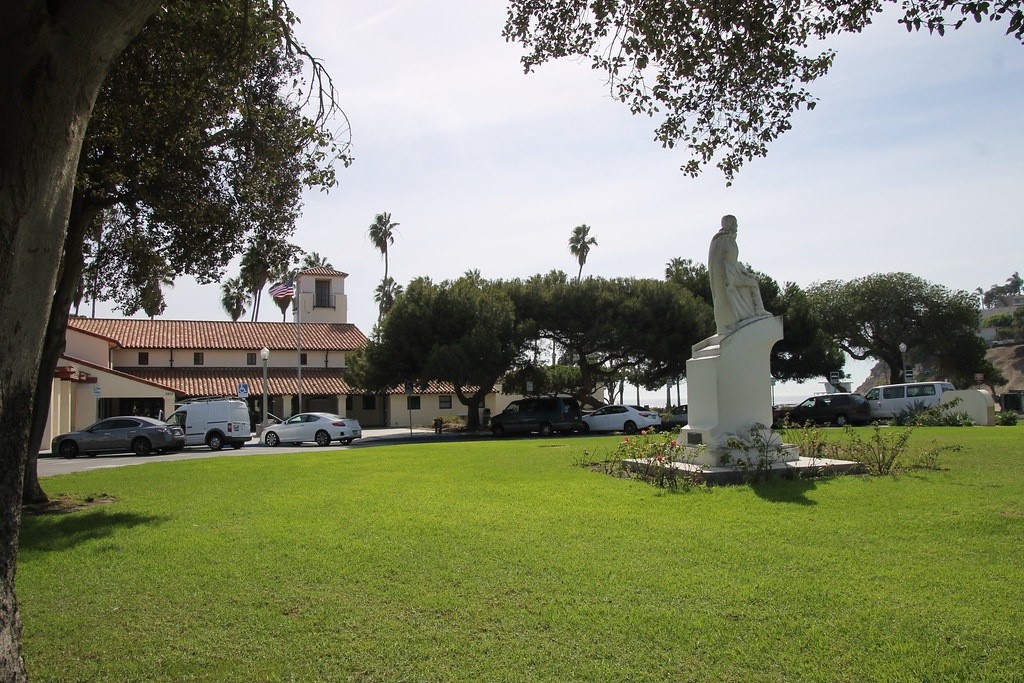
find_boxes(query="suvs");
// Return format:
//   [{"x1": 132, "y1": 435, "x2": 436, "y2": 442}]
[{"x1": 491, "y1": 397, "x2": 578, "y2": 436}]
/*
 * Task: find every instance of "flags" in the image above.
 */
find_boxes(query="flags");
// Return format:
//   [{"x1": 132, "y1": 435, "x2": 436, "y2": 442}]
[{"x1": 269, "y1": 277, "x2": 295, "y2": 297}]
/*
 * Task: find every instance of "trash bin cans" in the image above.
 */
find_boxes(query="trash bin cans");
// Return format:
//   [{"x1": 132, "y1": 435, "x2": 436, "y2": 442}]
[
  {"x1": 1000, "y1": 393, "x2": 1024, "y2": 413},
  {"x1": 478, "y1": 408, "x2": 491, "y2": 425}
]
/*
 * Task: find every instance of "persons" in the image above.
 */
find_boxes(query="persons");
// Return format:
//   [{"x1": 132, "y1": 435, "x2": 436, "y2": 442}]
[
  {"x1": 434, "y1": 417, "x2": 443, "y2": 434},
  {"x1": 708, "y1": 215, "x2": 772, "y2": 327}
]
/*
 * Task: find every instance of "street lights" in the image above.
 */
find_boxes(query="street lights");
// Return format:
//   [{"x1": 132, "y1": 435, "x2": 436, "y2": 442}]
[
  {"x1": 260, "y1": 347, "x2": 270, "y2": 426},
  {"x1": 293, "y1": 268, "x2": 301, "y2": 412},
  {"x1": 899, "y1": 343, "x2": 907, "y2": 382}
]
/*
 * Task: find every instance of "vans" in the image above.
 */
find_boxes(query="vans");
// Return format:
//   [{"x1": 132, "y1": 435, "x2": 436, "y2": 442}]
[
  {"x1": 159, "y1": 395, "x2": 252, "y2": 451},
  {"x1": 864, "y1": 381, "x2": 955, "y2": 421}
]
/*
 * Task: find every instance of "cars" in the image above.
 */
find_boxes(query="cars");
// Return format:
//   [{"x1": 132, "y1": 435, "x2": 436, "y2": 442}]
[
  {"x1": 786, "y1": 392, "x2": 870, "y2": 427},
  {"x1": 579, "y1": 405, "x2": 662, "y2": 434},
  {"x1": 51, "y1": 415, "x2": 186, "y2": 459},
  {"x1": 260, "y1": 412, "x2": 362, "y2": 446},
  {"x1": 773, "y1": 407, "x2": 785, "y2": 425},
  {"x1": 654, "y1": 405, "x2": 688, "y2": 435}
]
